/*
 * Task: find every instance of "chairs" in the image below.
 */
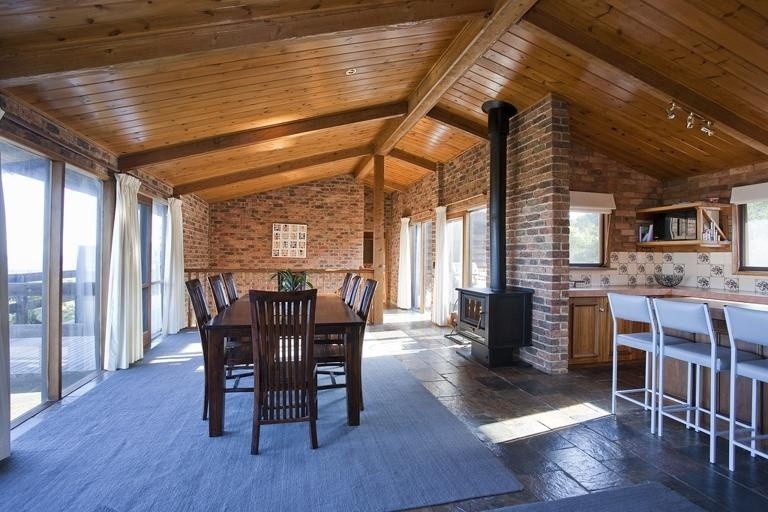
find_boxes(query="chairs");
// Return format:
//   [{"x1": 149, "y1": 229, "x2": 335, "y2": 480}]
[
  {"x1": 185, "y1": 272, "x2": 378, "y2": 455},
  {"x1": 607, "y1": 291, "x2": 768, "y2": 473}
]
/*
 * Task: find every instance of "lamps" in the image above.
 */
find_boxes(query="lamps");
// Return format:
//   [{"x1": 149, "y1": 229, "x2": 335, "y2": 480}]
[{"x1": 665, "y1": 100, "x2": 714, "y2": 136}]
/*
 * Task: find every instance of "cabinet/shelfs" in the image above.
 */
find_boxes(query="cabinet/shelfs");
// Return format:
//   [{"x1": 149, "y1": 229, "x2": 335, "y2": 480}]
[{"x1": 568, "y1": 297, "x2": 646, "y2": 368}]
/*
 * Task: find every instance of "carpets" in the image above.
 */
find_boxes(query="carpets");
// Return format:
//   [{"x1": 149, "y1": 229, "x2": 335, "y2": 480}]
[
  {"x1": 489, "y1": 479, "x2": 712, "y2": 511},
  {"x1": 0, "y1": 331, "x2": 522, "y2": 511}
]
